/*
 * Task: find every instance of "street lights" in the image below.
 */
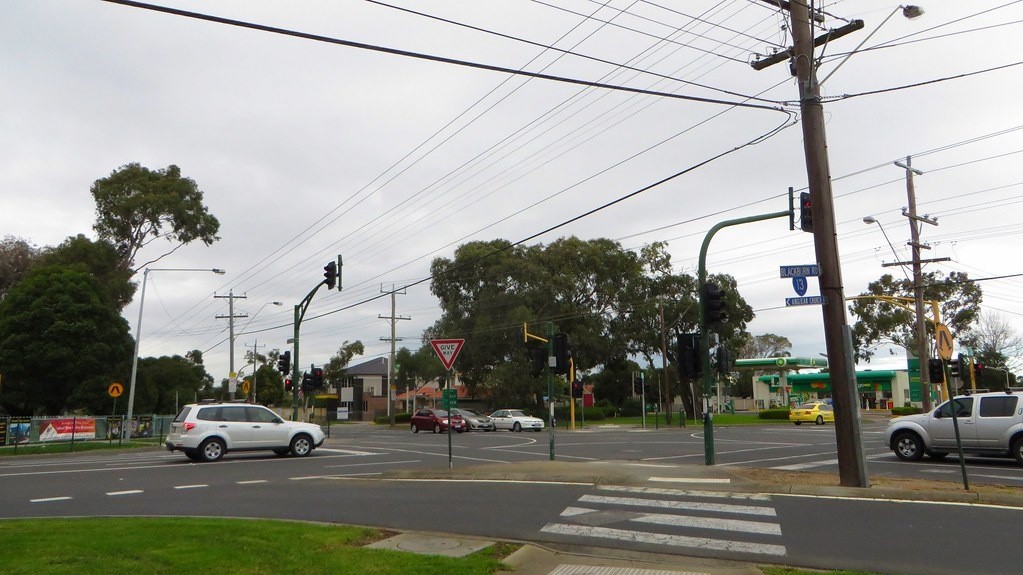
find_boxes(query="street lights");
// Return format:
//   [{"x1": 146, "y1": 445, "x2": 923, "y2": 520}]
[
  {"x1": 125, "y1": 267, "x2": 228, "y2": 443},
  {"x1": 862, "y1": 216, "x2": 933, "y2": 414},
  {"x1": 230, "y1": 301, "x2": 283, "y2": 402},
  {"x1": 797, "y1": 3, "x2": 923, "y2": 490}
]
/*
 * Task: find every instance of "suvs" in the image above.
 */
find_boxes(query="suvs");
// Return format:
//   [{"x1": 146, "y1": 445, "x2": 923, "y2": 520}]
[
  {"x1": 164, "y1": 398, "x2": 327, "y2": 463},
  {"x1": 881, "y1": 387, "x2": 1023, "y2": 466}
]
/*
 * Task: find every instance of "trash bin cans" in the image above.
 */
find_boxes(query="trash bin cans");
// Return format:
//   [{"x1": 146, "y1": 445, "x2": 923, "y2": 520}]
[
  {"x1": 701, "y1": 413, "x2": 706, "y2": 423},
  {"x1": 888, "y1": 401, "x2": 893, "y2": 408}
]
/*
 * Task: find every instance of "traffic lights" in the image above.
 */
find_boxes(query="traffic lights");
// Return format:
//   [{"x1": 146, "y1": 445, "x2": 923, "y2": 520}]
[
  {"x1": 278, "y1": 351, "x2": 290, "y2": 375},
  {"x1": 974, "y1": 362, "x2": 983, "y2": 369},
  {"x1": 800, "y1": 191, "x2": 812, "y2": 233},
  {"x1": 284, "y1": 363, "x2": 323, "y2": 392},
  {"x1": 323, "y1": 261, "x2": 337, "y2": 290},
  {"x1": 573, "y1": 382, "x2": 579, "y2": 398},
  {"x1": 554, "y1": 333, "x2": 573, "y2": 376},
  {"x1": 645, "y1": 384, "x2": 650, "y2": 392},
  {"x1": 703, "y1": 282, "x2": 726, "y2": 323},
  {"x1": 634, "y1": 377, "x2": 642, "y2": 396}
]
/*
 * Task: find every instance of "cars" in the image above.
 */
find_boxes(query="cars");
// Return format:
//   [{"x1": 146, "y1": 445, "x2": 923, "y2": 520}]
[
  {"x1": 487, "y1": 408, "x2": 545, "y2": 433},
  {"x1": 410, "y1": 408, "x2": 467, "y2": 434},
  {"x1": 447, "y1": 407, "x2": 495, "y2": 433},
  {"x1": 788, "y1": 402, "x2": 835, "y2": 426}
]
